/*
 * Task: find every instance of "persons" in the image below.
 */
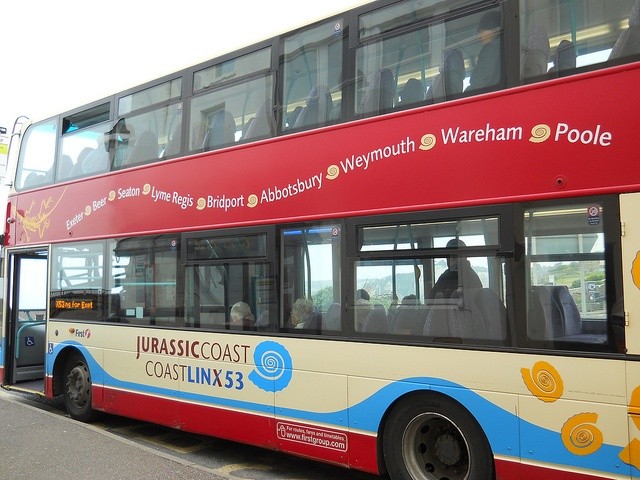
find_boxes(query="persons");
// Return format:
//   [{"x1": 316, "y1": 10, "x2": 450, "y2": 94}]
[
  {"x1": 222, "y1": 301, "x2": 255, "y2": 326},
  {"x1": 463, "y1": 10, "x2": 502, "y2": 93},
  {"x1": 356, "y1": 288, "x2": 370, "y2": 302},
  {"x1": 288, "y1": 298, "x2": 316, "y2": 330},
  {"x1": 388, "y1": 293, "x2": 421, "y2": 328},
  {"x1": 326, "y1": 68, "x2": 368, "y2": 121},
  {"x1": 426, "y1": 238, "x2": 484, "y2": 312}
]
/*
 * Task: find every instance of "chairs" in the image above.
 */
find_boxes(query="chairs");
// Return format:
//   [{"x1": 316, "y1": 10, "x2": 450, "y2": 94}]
[
  {"x1": 423, "y1": 292, "x2": 452, "y2": 337},
  {"x1": 361, "y1": 303, "x2": 390, "y2": 333},
  {"x1": 255, "y1": 311, "x2": 275, "y2": 328},
  {"x1": 470, "y1": 288, "x2": 509, "y2": 339},
  {"x1": 609, "y1": 7, "x2": 637, "y2": 62},
  {"x1": 201, "y1": 111, "x2": 236, "y2": 150},
  {"x1": 304, "y1": 312, "x2": 322, "y2": 329},
  {"x1": 244, "y1": 99, "x2": 274, "y2": 137},
  {"x1": 425, "y1": 49, "x2": 466, "y2": 100},
  {"x1": 528, "y1": 286, "x2": 603, "y2": 344},
  {"x1": 359, "y1": 70, "x2": 397, "y2": 114},
  {"x1": 392, "y1": 295, "x2": 416, "y2": 334},
  {"x1": 135, "y1": 131, "x2": 158, "y2": 161},
  {"x1": 326, "y1": 303, "x2": 342, "y2": 331},
  {"x1": 450, "y1": 292, "x2": 486, "y2": 340},
  {"x1": 357, "y1": 299, "x2": 372, "y2": 330},
  {"x1": 396, "y1": 80, "x2": 425, "y2": 105},
  {"x1": 291, "y1": 85, "x2": 333, "y2": 127},
  {"x1": 552, "y1": 278, "x2": 607, "y2": 340},
  {"x1": 547, "y1": 40, "x2": 575, "y2": 72},
  {"x1": 520, "y1": 51, "x2": 547, "y2": 76}
]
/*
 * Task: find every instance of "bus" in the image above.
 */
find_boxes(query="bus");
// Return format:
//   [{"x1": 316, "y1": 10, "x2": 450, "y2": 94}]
[{"x1": 0, "y1": 2, "x2": 640, "y2": 480}]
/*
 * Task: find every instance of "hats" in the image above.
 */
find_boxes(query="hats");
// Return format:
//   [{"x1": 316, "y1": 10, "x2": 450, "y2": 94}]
[{"x1": 477, "y1": 12, "x2": 501, "y2": 30}]
